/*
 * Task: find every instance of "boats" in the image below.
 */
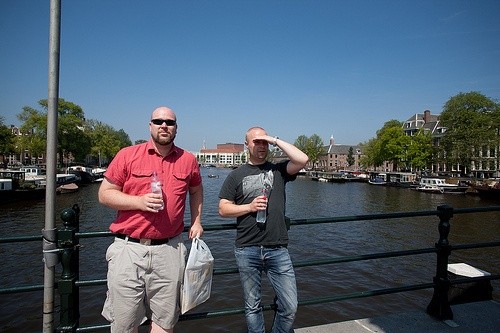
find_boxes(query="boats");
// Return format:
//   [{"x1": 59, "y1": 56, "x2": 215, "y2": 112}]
[
  {"x1": 418, "y1": 185, "x2": 445, "y2": 194},
  {"x1": 0, "y1": 164, "x2": 107, "y2": 202},
  {"x1": 368, "y1": 178, "x2": 388, "y2": 185},
  {"x1": 208, "y1": 173, "x2": 220, "y2": 179}
]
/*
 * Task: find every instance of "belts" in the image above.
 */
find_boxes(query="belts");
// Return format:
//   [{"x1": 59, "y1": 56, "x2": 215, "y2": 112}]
[{"x1": 115, "y1": 232, "x2": 180, "y2": 245}]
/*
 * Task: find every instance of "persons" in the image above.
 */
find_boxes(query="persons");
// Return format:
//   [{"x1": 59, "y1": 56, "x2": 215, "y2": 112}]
[
  {"x1": 218, "y1": 126, "x2": 310, "y2": 333},
  {"x1": 98, "y1": 106, "x2": 205, "y2": 333}
]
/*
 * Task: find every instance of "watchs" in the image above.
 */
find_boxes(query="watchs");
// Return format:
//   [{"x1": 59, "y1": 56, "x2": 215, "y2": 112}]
[{"x1": 273, "y1": 136, "x2": 280, "y2": 147}]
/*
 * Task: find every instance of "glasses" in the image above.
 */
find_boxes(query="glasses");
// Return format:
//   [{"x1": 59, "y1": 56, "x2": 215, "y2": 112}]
[{"x1": 150, "y1": 119, "x2": 176, "y2": 126}]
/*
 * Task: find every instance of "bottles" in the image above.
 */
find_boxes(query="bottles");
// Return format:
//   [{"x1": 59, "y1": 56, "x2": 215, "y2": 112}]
[
  {"x1": 149, "y1": 171, "x2": 165, "y2": 210},
  {"x1": 256, "y1": 188, "x2": 269, "y2": 223}
]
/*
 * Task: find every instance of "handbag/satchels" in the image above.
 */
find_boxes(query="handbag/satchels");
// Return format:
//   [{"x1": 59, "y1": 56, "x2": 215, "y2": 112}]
[{"x1": 180, "y1": 236, "x2": 215, "y2": 315}]
